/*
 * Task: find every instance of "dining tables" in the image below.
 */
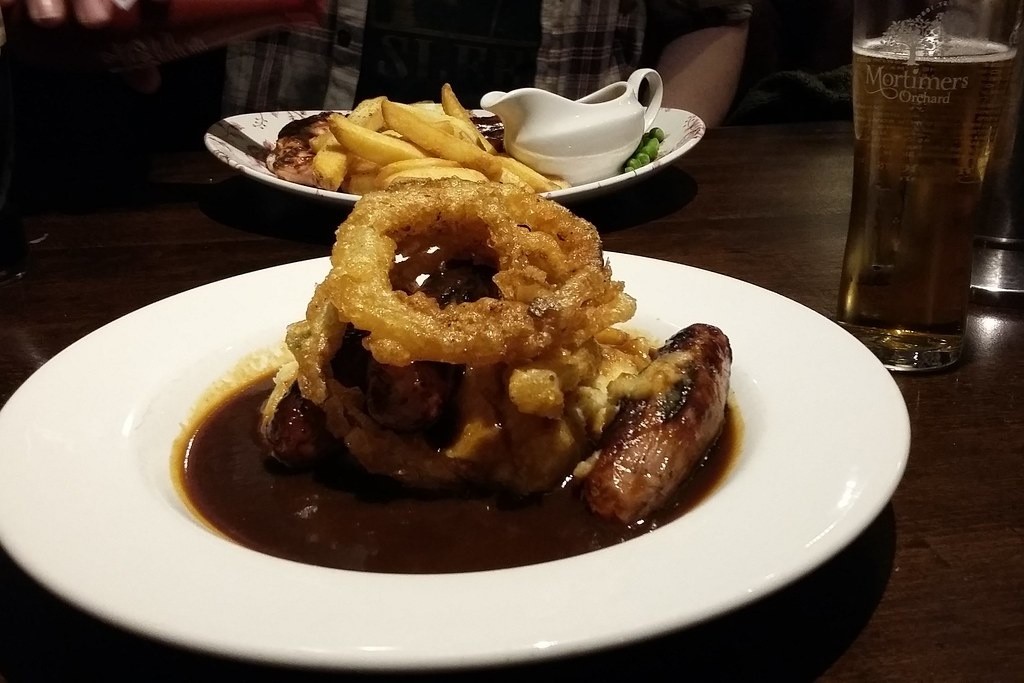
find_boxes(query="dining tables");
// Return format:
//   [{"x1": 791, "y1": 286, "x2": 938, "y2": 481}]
[{"x1": 0, "y1": 114, "x2": 1022, "y2": 683}]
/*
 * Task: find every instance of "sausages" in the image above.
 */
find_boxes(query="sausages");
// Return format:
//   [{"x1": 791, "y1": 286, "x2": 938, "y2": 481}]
[{"x1": 583, "y1": 320, "x2": 732, "y2": 525}]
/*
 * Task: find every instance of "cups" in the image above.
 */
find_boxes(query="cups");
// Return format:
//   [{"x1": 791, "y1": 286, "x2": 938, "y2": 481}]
[{"x1": 837, "y1": 1, "x2": 1023, "y2": 374}]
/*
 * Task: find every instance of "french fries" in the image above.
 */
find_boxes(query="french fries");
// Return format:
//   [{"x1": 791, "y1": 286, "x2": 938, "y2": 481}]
[{"x1": 313, "y1": 90, "x2": 567, "y2": 196}]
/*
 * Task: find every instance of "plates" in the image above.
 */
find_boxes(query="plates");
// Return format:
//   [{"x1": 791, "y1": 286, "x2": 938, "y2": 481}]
[
  {"x1": 207, "y1": 105, "x2": 707, "y2": 208},
  {"x1": 1, "y1": 249, "x2": 915, "y2": 671}
]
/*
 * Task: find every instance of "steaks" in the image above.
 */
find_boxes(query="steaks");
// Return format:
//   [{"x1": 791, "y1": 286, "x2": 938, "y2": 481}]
[{"x1": 266, "y1": 114, "x2": 331, "y2": 184}]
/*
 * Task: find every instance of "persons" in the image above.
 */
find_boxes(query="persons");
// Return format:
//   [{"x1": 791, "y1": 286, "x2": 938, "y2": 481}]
[{"x1": 0, "y1": 1, "x2": 749, "y2": 196}]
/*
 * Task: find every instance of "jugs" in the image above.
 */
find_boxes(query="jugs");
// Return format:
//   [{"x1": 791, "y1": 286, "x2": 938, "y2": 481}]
[{"x1": 481, "y1": 66, "x2": 663, "y2": 188}]
